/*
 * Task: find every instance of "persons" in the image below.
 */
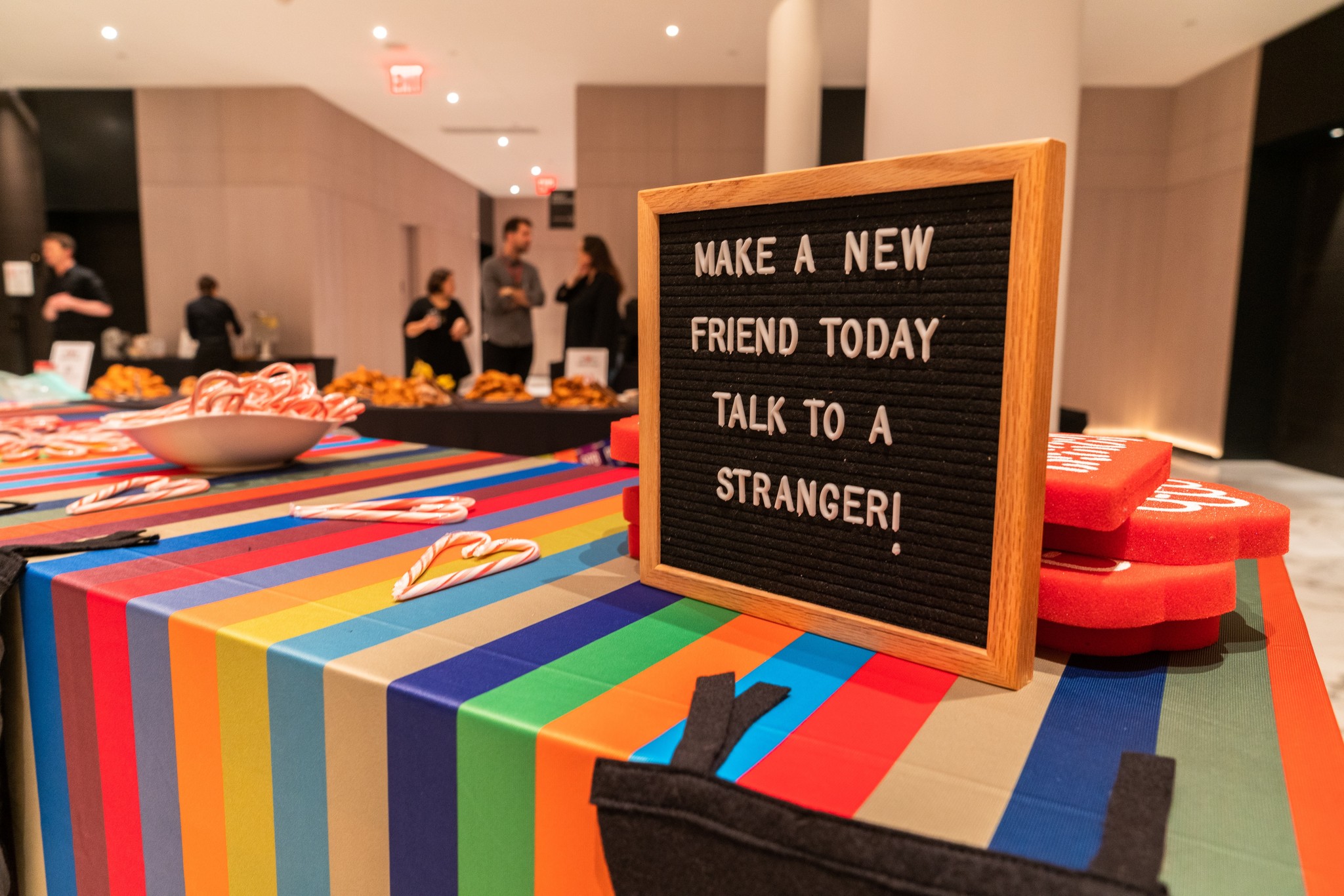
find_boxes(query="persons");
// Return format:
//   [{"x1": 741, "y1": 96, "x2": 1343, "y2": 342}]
[
  {"x1": 184, "y1": 276, "x2": 243, "y2": 371},
  {"x1": 554, "y1": 235, "x2": 625, "y2": 373},
  {"x1": 38, "y1": 234, "x2": 112, "y2": 382},
  {"x1": 404, "y1": 269, "x2": 472, "y2": 392},
  {"x1": 617, "y1": 300, "x2": 638, "y2": 393},
  {"x1": 482, "y1": 220, "x2": 544, "y2": 382}
]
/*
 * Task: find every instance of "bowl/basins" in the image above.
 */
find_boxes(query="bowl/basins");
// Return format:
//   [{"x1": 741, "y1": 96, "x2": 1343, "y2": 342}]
[{"x1": 101, "y1": 413, "x2": 357, "y2": 472}]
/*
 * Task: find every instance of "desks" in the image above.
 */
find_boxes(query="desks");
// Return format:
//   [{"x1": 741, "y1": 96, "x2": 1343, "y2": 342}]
[
  {"x1": 344, "y1": 398, "x2": 636, "y2": 457},
  {"x1": 94, "y1": 356, "x2": 337, "y2": 393},
  {"x1": 0, "y1": 403, "x2": 1344, "y2": 896}
]
